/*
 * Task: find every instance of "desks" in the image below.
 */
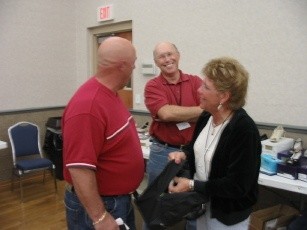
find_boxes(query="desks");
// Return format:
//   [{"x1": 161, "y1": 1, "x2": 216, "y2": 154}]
[
  {"x1": 43, "y1": 126, "x2": 64, "y2": 181},
  {"x1": 258, "y1": 172, "x2": 307, "y2": 213}
]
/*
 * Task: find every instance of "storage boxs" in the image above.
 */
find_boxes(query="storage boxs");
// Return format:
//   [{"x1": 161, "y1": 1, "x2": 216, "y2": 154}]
[{"x1": 249, "y1": 202, "x2": 298, "y2": 230}]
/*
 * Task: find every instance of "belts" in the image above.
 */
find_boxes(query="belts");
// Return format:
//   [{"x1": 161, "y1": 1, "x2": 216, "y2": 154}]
[
  {"x1": 71, "y1": 186, "x2": 133, "y2": 200},
  {"x1": 155, "y1": 137, "x2": 185, "y2": 152}
]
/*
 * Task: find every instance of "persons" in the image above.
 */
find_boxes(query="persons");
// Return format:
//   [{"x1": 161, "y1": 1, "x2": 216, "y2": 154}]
[
  {"x1": 61, "y1": 35, "x2": 150, "y2": 230},
  {"x1": 167, "y1": 57, "x2": 262, "y2": 230},
  {"x1": 144, "y1": 41, "x2": 204, "y2": 230}
]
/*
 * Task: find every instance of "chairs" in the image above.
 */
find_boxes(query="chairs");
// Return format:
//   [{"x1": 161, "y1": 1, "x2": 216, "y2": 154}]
[{"x1": 7, "y1": 121, "x2": 58, "y2": 203}]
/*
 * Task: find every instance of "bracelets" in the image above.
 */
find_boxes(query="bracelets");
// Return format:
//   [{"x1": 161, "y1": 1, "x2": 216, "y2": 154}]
[
  {"x1": 92, "y1": 209, "x2": 108, "y2": 226},
  {"x1": 189, "y1": 179, "x2": 195, "y2": 192}
]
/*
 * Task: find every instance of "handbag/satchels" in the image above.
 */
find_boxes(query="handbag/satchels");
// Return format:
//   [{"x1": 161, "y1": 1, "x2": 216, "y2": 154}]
[{"x1": 134, "y1": 158, "x2": 209, "y2": 230}]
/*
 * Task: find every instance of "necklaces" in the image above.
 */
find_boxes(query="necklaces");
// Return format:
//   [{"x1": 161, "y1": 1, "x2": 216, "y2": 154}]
[{"x1": 202, "y1": 111, "x2": 234, "y2": 181}]
